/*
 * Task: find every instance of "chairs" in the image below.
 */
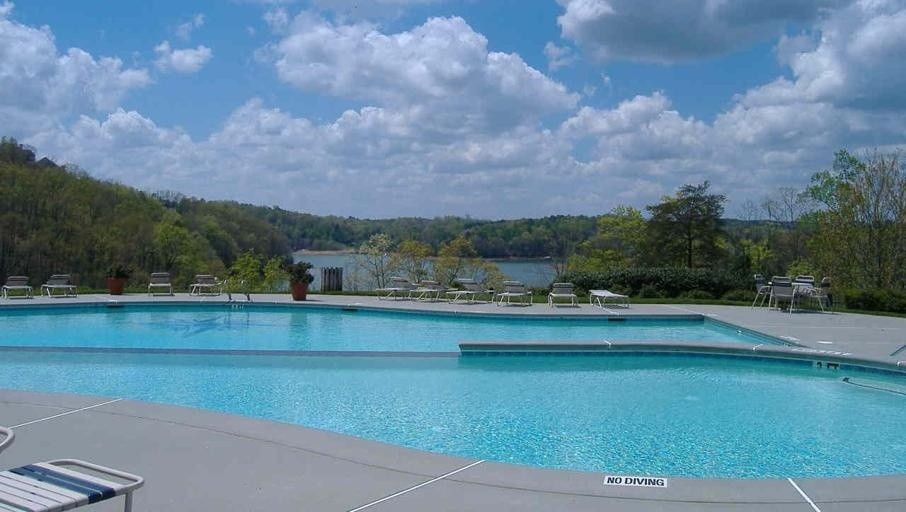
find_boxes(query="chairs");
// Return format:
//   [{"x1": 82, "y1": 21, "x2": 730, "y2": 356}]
[
  {"x1": 1, "y1": 274, "x2": 79, "y2": 301},
  {"x1": 0, "y1": 425, "x2": 144, "y2": 512},
  {"x1": 376, "y1": 276, "x2": 630, "y2": 311},
  {"x1": 147, "y1": 272, "x2": 223, "y2": 296},
  {"x1": 750, "y1": 273, "x2": 834, "y2": 315}
]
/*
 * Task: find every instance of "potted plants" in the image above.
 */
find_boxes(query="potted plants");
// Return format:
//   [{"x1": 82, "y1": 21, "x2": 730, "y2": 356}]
[
  {"x1": 285, "y1": 261, "x2": 314, "y2": 300},
  {"x1": 104, "y1": 264, "x2": 130, "y2": 295}
]
